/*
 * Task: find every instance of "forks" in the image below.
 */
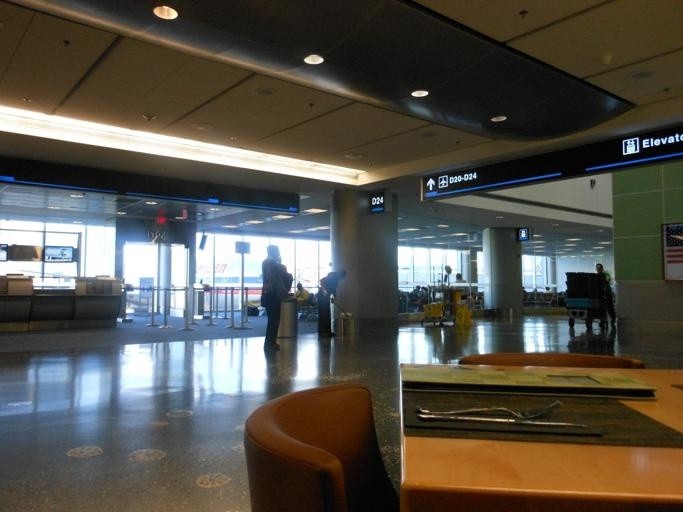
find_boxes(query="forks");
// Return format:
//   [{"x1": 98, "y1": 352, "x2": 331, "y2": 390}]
[{"x1": 417, "y1": 401, "x2": 564, "y2": 420}]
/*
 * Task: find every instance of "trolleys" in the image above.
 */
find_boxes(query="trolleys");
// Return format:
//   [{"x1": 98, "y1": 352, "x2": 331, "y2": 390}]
[
  {"x1": 565, "y1": 328, "x2": 615, "y2": 356},
  {"x1": 564, "y1": 277, "x2": 610, "y2": 333},
  {"x1": 419, "y1": 284, "x2": 471, "y2": 327}
]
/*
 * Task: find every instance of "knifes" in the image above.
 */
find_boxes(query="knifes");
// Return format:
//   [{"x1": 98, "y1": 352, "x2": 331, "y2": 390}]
[{"x1": 418, "y1": 414, "x2": 588, "y2": 428}]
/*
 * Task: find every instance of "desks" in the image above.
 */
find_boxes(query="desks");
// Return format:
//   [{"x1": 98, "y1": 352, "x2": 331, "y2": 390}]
[{"x1": 400, "y1": 364, "x2": 683, "y2": 511}]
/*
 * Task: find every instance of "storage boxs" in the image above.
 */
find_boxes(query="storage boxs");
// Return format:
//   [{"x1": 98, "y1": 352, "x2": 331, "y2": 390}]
[{"x1": 422, "y1": 302, "x2": 444, "y2": 327}]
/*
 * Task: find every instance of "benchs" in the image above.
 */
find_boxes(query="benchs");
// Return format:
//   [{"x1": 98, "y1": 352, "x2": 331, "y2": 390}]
[{"x1": 523, "y1": 291, "x2": 554, "y2": 307}]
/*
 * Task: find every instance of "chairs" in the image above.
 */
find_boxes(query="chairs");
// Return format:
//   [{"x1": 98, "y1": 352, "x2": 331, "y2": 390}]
[
  {"x1": 459, "y1": 352, "x2": 646, "y2": 369},
  {"x1": 467, "y1": 296, "x2": 483, "y2": 310},
  {"x1": 298, "y1": 293, "x2": 319, "y2": 321},
  {"x1": 244, "y1": 384, "x2": 400, "y2": 512}
]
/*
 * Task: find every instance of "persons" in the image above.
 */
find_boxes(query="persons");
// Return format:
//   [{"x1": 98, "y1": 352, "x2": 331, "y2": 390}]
[
  {"x1": 455, "y1": 274, "x2": 467, "y2": 299},
  {"x1": 261, "y1": 245, "x2": 289, "y2": 351},
  {"x1": 318, "y1": 270, "x2": 346, "y2": 337},
  {"x1": 296, "y1": 284, "x2": 310, "y2": 309},
  {"x1": 414, "y1": 286, "x2": 432, "y2": 304},
  {"x1": 596, "y1": 264, "x2": 617, "y2": 328},
  {"x1": 544, "y1": 287, "x2": 555, "y2": 301}
]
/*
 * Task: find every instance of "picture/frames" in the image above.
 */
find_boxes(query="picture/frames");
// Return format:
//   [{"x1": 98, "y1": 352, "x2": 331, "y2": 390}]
[{"x1": 661, "y1": 222, "x2": 683, "y2": 282}]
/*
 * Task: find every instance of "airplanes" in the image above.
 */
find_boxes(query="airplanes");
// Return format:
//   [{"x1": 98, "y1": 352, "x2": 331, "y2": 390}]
[{"x1": 171, "y1": 254, "x2": 267, "y2": 312}]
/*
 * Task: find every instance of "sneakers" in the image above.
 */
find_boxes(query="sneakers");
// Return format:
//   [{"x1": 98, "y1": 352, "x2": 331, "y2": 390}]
[
  {"x1": 264, "y1": 343, "x2": 280, "y2": 350},
  {"x1": 319, "y1": 332, "x2": 336, "y2": 337}
]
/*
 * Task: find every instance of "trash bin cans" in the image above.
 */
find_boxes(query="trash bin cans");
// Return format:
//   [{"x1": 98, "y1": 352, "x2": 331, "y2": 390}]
[{"x1": 277, "y1": 299, "x2": 298, "y2": 338}]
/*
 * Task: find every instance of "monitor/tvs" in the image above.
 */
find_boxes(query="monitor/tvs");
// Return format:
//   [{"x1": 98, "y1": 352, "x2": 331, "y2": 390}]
[
  {"x1": 45, "y1": 246, "x2": 73, "y2": 263},
  {"x1": 0, "y1": 244, "x2": 8, "y2": 262}
]
